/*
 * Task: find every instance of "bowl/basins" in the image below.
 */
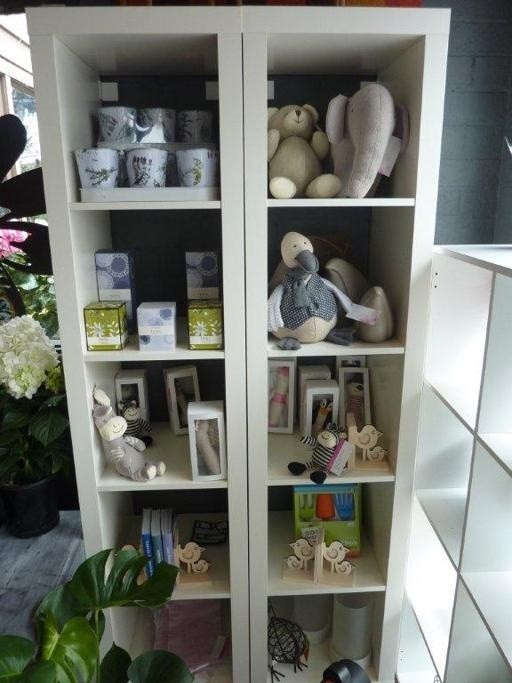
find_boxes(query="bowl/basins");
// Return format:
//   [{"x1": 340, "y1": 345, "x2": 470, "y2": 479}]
[
  {"x1": 334, "y1": 649, "x2": 372, "y2": 671},
  {"x1": 291, "y1": 621, "x2": 330, "y2": 645}
]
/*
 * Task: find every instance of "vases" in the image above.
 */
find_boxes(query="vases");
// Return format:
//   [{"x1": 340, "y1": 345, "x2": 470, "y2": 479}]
[{"x1": 1, "y1": 467, "x2": 60, "y2": 537}]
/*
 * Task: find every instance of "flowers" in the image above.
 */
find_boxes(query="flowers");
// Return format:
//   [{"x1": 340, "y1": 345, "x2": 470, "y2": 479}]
[{"x1": 0, "y1": 219, "x2": 53, "y2": 478}]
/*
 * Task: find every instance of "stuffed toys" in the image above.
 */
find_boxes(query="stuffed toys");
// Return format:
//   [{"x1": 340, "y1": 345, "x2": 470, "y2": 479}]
[
  {"x1": 267, "y1": 81, "x2": 394, "y2": 485},
  {"x1": 93, "y1": 364, "x2": 227, "y2": 484}
]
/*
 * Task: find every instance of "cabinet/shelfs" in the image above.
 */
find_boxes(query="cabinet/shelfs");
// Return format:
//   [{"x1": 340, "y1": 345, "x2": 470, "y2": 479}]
[
  {"x1": 392, "y1": 233, "x2": 512, "y2": 682},
  {"x1": 26, "y1": 1, "x2": 245, "y2": 678},
  {"x1": 247, "y1": 6, "x2": 454, "y2": 681}
]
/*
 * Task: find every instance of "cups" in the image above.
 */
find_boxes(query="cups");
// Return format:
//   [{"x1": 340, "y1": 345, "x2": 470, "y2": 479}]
[
  {"x1": 332, "y1": 594, "x2": 374, "y2": 659},
  {"x1": 95, "y1": 104, "x2": 137, "y2": 144},
  {"x1": 137, "y1": 108, "x2": 177, "y2": 143},
  {"x1": 124, "y1": 146, "x2": 169, "y2": 187},
  {"x1": 177, "y1": 108, "x2": 214, "y2": 143},
  {"x1": 71, "y1": 148, "x2": 121, "y2": 189},
  {"x1": 174, "y1": 147, "x2": 216, "y2": 188}
]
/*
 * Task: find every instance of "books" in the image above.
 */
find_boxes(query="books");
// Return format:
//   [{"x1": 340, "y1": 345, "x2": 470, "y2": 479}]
[{"x1": 141, "y1": 507, "x2": 180, "y2": 580}]
